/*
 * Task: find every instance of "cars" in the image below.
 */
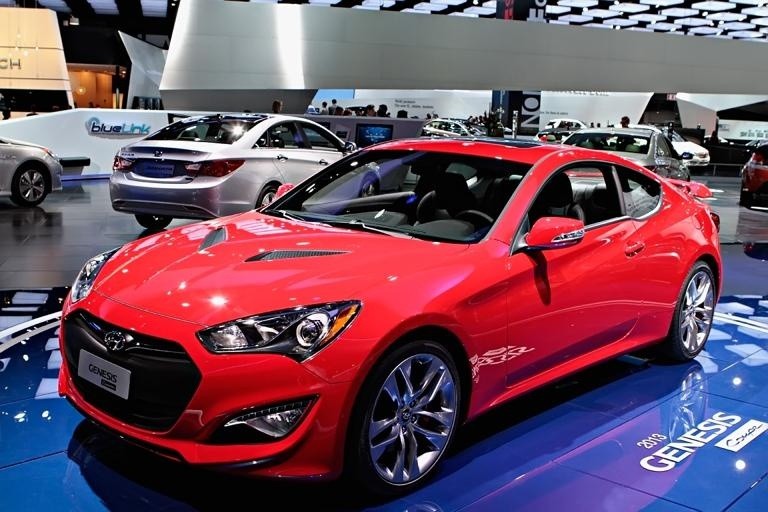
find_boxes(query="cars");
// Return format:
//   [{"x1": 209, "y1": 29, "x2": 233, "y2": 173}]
[
  {"x1": 54, "y1": 137, "x2": 724, "y2": 492},
  {"x1": 391, "y1": 359, "x2": 710, "y2": 509},
  {"x1": 424, "y1": 117, "x2": 712, "y2": 199},
  {"x1": 703, "y1": 134, "x2": 746, "y2": 159},
  {"x1": 739, "y1": 140, "x2": 767, "y2": 262},
  {"x1": 0, "y1": 135, "x2": 64, "y2": 207},
  {"x1": 108, "y1": 110, "x2": 381, "y2": 229}
]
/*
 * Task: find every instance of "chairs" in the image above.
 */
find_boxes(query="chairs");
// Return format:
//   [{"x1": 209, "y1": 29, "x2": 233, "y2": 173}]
[
  {"x1": 480, "y1": 172, "x2": 609, "y2": 224},
  {"x1": 411, "y1": 172, "x2": 474, "y2": 223}
]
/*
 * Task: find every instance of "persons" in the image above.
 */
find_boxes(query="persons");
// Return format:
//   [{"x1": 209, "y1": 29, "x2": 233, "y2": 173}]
[
  {"x1": 272, "y1": 100, "x2": 283, "y2": 113},
  {"x1": 616, "y1": 116, "x2": 636, "y2": 151},
  {"x1": 397, "y1": 110, "x2": 408, "y2": 118},
  {"x1": 319, "y1": 99, "x2": 390, "y2": 118}
]
